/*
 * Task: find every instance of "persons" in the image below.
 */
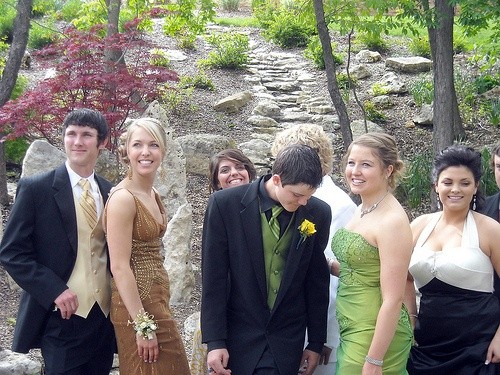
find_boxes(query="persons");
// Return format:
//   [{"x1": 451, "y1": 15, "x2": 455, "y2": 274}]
[
  {"x1": 0, "y1": 108, "x2": 118, "y2": 375},
  {"x1": 272, "y1": 123, "x2": 358, "y2": 375},
  {"x1": 102, "y1": 117, "x2": 190, "y2": 375},
  {"x1": 326, "y1": 133, "x2": 413, "y2": 375},
  {"x1": 209, "y1": 150, "x2": 257, "y2": 193},
  {"x1": 476, "y1": 144, "x2": 500, "y2": 223},
  {"x1": 404, "y1": 145, "x2": 500, "y2": 375},
  {"x1": 201, "y1": 145, "x2": 332, "y2": 375}
]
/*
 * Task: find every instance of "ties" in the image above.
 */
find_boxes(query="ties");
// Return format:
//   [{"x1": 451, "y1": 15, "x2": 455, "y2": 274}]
[
  {"x1": 77, "y1": 177, "x2": 100, "y2": 230},
  {"x1": 267, "y1": 204, "x2": 286, "y2": 240}
]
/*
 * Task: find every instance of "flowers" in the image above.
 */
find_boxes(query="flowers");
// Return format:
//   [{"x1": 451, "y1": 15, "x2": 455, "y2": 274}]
[{"x1": 295, "y1": 219, "x2": 317, "y2": 251}]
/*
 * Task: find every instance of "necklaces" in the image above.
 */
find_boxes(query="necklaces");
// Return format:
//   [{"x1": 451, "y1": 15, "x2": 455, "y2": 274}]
[{"x1": 360, "y1": 188, "x2": 388, "y2": 218}]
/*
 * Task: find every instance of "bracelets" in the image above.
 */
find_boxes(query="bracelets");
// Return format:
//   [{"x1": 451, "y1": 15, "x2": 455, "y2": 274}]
[
  {"x1": 366, "y1": 356, "x2": 383, "y2": 366},
  {"x1": 327, "y1": 258, "x2": 334, "y2": 269},
  {"x1": 409, "y1": 314, "x2": 417, "y2": 318},
  {"x1": 133, "y1": 312, "x2": 158, "y2": 340}
]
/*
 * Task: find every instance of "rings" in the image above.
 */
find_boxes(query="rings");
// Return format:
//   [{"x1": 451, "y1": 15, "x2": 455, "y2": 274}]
[{"x1": 206, "y1": 368, "x2": 212, "y2": 373}]
